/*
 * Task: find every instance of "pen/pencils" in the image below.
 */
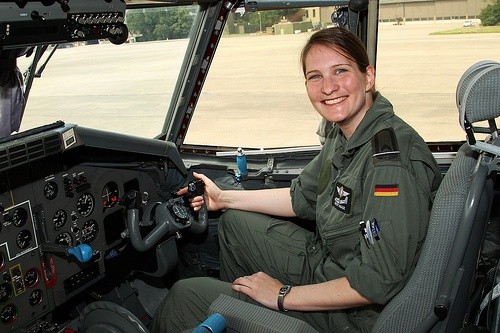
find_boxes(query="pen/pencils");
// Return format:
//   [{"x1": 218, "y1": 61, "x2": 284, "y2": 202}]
[
  {"x1": 371, "y1": 218, "x2": 382, "y2": 241},
  {"x1": 358, "y1": 221, "x2": 370, "y2": 247},
  {"x1": 366, "y1": 219, "x2": 374, "y2": 244}
]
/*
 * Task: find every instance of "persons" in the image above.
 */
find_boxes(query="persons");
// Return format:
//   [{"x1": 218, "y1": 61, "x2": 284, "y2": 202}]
[{"x1": 159, "y1": 27, "x2": 439, "y2": 333}]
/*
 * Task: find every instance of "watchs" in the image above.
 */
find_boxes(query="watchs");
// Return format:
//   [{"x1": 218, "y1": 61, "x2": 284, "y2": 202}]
[{"x1": 278, "y1": 285, "x2": 292, "y2": 313}]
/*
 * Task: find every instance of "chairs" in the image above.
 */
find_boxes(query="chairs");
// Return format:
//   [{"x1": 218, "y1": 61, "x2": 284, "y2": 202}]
[{"x1": 207, "y1": 60, "x2": 500, "y2": 333}]
